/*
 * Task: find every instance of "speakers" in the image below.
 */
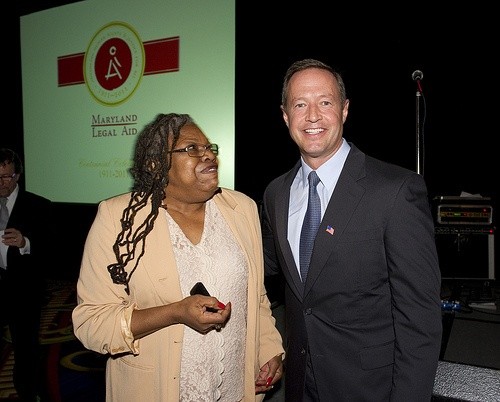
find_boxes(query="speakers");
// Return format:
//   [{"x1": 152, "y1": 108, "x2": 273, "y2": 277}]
[{"x1": 430, "y1": 224, "x2": 494, "y2": 281}]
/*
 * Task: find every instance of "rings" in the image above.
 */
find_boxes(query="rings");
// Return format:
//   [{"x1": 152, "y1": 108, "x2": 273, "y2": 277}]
[
  {"x1": 14, "y1": 238, "x2": 16, "y2": 242},
  {"x1": 215, "y1": 324, "x2": 220, "y2": 329}
]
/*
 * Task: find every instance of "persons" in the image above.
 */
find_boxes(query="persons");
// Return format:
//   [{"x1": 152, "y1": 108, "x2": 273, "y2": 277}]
[
  {"x1": 260, "y1": 59, "x2": 442, "y2": 402},
  {"x1": 0, "y1": 147, "x2": 65, "y2": 402},
  {"x1": 71, "y1": 111, "x2": 287, "y2": 402}
]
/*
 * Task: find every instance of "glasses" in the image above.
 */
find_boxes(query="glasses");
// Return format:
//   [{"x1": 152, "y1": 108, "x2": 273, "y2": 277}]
[
  {"x1": 168, "y1": 144, "x2": 219, "y2": 157},
  {"x1": 1, "y1": 173, "x2": 15, "y2": 182}
]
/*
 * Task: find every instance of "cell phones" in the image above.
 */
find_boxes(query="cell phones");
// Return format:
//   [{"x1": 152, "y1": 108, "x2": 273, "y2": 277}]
[{"x1": 190, "y1": 282, "x2": 218, "y2": 314}]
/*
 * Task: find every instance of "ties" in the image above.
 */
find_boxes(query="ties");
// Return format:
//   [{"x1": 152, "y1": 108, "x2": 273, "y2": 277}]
[
  {"x1": 300, "y1": 171, "x2": 321, "y2": 283},
  {"x1": 0, "y1": 197, "x2": 9, "y2": 231}
]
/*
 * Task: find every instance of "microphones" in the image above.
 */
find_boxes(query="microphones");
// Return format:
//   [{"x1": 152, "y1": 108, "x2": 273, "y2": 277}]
[{"x1": 412, "y1": 70, "x2": 424, "y2": 94}]
